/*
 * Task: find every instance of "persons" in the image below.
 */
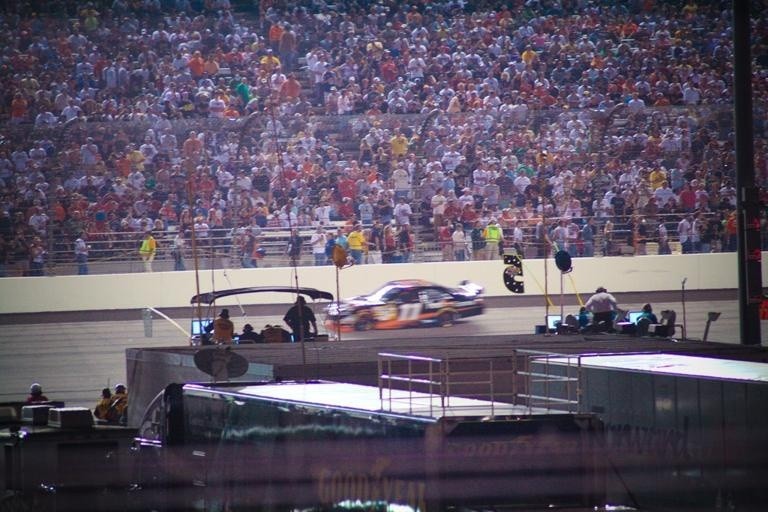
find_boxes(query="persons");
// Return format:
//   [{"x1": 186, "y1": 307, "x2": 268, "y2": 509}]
[
  {"x1": 93, "y1": 388, "x2": 118, "y2": 422},
  {"x1": 112, "y1": 383, "x2": 128, "y2": 416},
  {"x1": 1, "y1": 1, "x2": 767, "y2": 277},
  {"x1": 26, "y1": 382, "x2": 49, "y2": 402},
  {"x1": 284, "y1": 296, "x2": 318, "y2": 343},
  {"x1": 204, "y1": 308, "x2": 235, "y2": 345},
  {"x1": 635, "y1": 303, "x2": 658, "y2": 331},
  {"x1": 583, "y1": 286, "x2": 619, "y2": 334},
  {"x1": 239, "y1": 324, "x2": 260, "y2": 342},
  {"x1": 255, "y1": 323, "x2": 291, "y2": 343},
  {"x1": 578, "y1": 306, "x2": 590, "y2": 328}
]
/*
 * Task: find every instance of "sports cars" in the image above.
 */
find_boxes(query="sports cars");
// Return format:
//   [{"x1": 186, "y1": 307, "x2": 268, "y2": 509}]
[{"x1": 325, "y1": 279, "x2": 486, "y2": 332}]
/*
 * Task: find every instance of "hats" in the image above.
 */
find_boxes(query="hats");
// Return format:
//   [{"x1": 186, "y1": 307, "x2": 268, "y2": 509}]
[
  {"x1": 116, "y1": 384, "x2": 128, "y2": 396},
  {"x1": 243, "y1": 323, "x2": 253, "y2": 333},
  {"x1": 28, "y1": 383, "x2": 43, "y2": 394},
  {"x1": 100, "y1": 388, "x2": 113, "y2": 399},
  {"x1": 296, "y1": 296, "x2": 307, "y2": 306},
  {"x1": 218, "y1": 309, "x2": 231, "y2": 318}
]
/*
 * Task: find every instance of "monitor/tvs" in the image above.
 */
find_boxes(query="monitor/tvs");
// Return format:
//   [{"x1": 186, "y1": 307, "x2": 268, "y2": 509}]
[
  {"x1": 575, "y1": 315, "x2": 580, "y2": 320},
  {"x1": 190, "y1": 319, "x2": 215, "y2": 338},
  {"x1": 291, "y1": 333, "x2": 295, "y2": 342},
  {"x1": 234, "y1": 335, "x2": 240, "y2": 345},
  {"x1": 545, "y1": 314, "x2": 561, "y2": 334},
  {"x1": 628, "y1": 311, "x2": 642, "y2": 326}
]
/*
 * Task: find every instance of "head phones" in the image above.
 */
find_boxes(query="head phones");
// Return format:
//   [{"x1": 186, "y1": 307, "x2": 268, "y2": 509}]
[{"x1": 243, "y1": 323, "x2": 254, "y2": 332}]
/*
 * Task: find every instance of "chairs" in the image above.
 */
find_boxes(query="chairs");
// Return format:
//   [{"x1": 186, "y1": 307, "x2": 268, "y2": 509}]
[
  {"x1": 637, "y1": 317, "x2": 652, "y2": 335},
  {"x1": 264, "y1": 328, "x2": 282, "y2": 343},
  {"x1": 565, "y1": 314, "x2": 579, "y2": 335},
  {"x1": 313, "y1": 334, "x2": 328, "y2": 341}
]
